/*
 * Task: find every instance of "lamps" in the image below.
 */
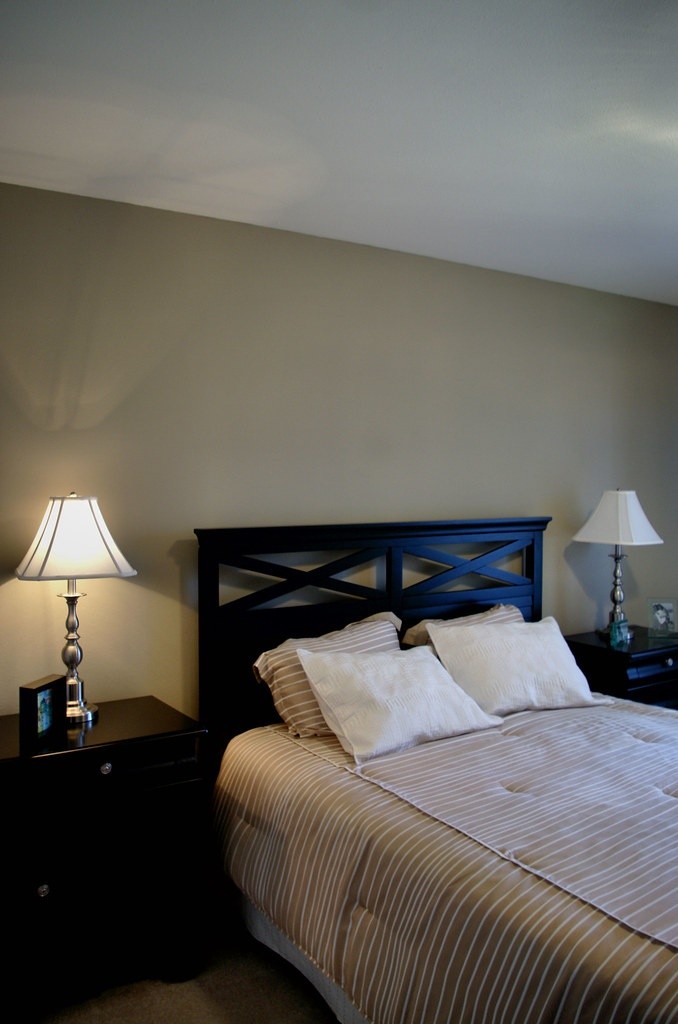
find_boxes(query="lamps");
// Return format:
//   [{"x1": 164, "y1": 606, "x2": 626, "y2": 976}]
[
  {"x1": 570, "y1": 486, "x2": 666, "y2": 640},
  {"x1": 15, "y1": 491, "x2": 140, "y2": 725}
]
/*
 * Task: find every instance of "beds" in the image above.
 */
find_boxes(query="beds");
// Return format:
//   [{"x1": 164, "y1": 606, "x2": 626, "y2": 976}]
[{"x1": 193, "y1": 517, "x2": 678, "y2": 1024}]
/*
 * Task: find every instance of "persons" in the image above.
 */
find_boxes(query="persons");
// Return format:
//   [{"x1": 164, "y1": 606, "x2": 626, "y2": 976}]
[
  {"x1": 617, "y1": 626, "x2": 623, "y2": 643},
  {"x1": 41, "y1": 699, "x2": 48, "y2": 730},
  {"x1": 654, "y1": 604, "x2": 668, "y2": 631}
]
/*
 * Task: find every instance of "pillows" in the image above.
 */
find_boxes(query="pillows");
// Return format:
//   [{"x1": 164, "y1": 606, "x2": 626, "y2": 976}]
[
  {"x1": 251, "y1": 611, "x2": 401, "y2": 737},
  {"x1": 296, "y1": 643, "x2": 507, "y2": 767},
  {"x1": 401, "y1": 605, "x2": 526, "y2": 664},
  {"x1": 424, "y1": 615, "x2": 615, "y2": 720}
]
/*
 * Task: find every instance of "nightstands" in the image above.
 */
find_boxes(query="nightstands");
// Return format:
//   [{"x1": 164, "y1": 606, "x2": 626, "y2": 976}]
[
  {"x1": 0, "y1": 695, "x2": 210, "y2": 990},
  {"x1": 561, "y1": 622, "x2": 678, "y2": 712}
]
